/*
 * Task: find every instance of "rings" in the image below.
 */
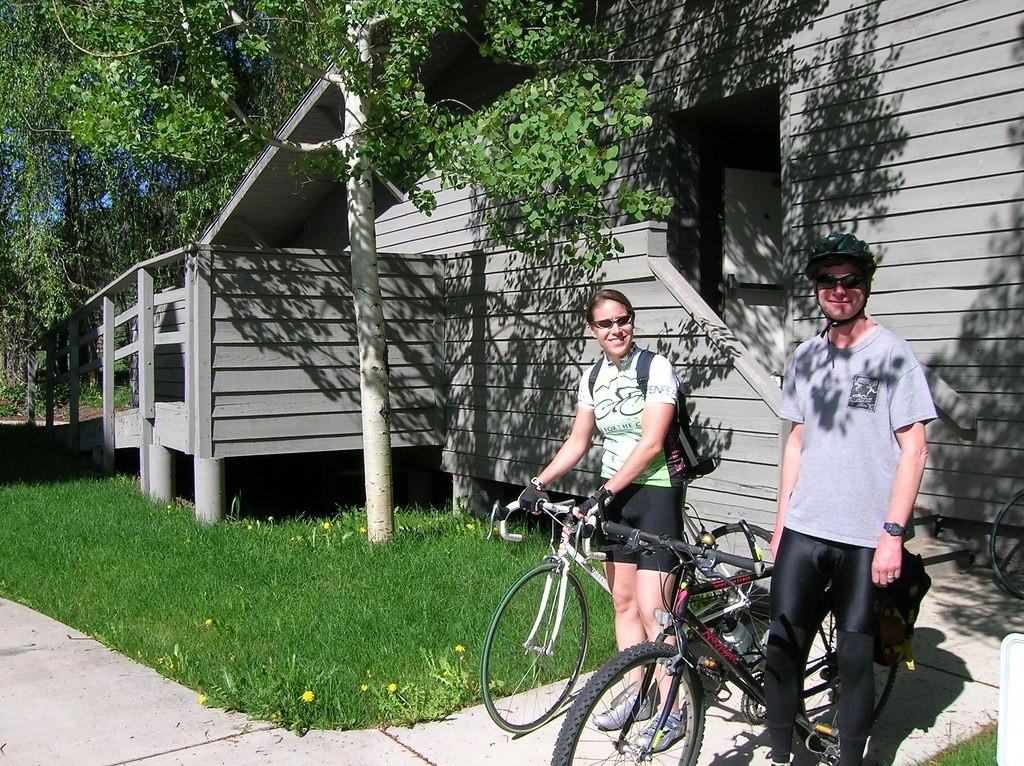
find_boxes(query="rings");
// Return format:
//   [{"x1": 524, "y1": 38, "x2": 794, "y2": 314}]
[{"x1": 888, "y1": 576, "x2": 893, "y2": 578}]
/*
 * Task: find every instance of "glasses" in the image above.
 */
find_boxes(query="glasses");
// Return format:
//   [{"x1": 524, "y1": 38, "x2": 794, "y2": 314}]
[
  {"x1": 816, "y1": 272, "x2": 867, "y2": 291},
  {"x1": 588, "y1": 315, "x2": 633, "y2": 329}
]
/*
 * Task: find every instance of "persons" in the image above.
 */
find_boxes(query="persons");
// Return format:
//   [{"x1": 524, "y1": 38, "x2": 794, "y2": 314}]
[
  {"x1": 518, "y1": 290, "x2": 689, "y2": 754},
  {"x1": 765, "y1": 231, "x2": 938, "y2": 766}
]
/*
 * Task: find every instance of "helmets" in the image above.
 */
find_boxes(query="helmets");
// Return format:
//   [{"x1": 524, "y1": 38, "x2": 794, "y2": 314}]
[{"x1": 806, "y1": 231, "x2": 877, "y2": 282}]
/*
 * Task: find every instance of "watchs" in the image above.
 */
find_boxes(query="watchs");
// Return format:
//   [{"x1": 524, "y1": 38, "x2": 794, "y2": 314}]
[{"x1": 883, "y1": 522, "x2": 906, "y2": 538}]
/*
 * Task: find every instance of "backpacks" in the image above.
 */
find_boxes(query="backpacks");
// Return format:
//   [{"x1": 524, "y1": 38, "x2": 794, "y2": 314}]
[
  {"x1": 676, "y1": 390, "x2": 718, "y2": 478},
  {"x1": 873, "y1": 546, "x2": 932, "y2": 671}
]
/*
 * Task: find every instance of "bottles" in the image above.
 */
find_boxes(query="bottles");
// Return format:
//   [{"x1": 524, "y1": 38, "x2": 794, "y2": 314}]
[
  {"x1": 759, "y1": 619, "x2": 771, "y2": 670},
  {"x1": 720, "y1": 616, "x2": 760, "y2": 664}
]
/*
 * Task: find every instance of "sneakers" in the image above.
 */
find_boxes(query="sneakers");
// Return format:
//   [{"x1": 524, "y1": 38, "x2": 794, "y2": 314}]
[
  {"x1": 593, "y1": 692, "x2": 651, "y2": 730},
  {"x1": 634, "y1": 709, "x2": 686, "y2": 752}
]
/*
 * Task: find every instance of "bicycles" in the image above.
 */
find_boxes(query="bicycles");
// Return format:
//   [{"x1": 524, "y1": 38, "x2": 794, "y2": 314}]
[
  {"x1": 480, "y1": 498, "x2": 773, "y2": 734},
  {"x1": 550, "y1": 489, "x2": 932, "y2": 766}
]
